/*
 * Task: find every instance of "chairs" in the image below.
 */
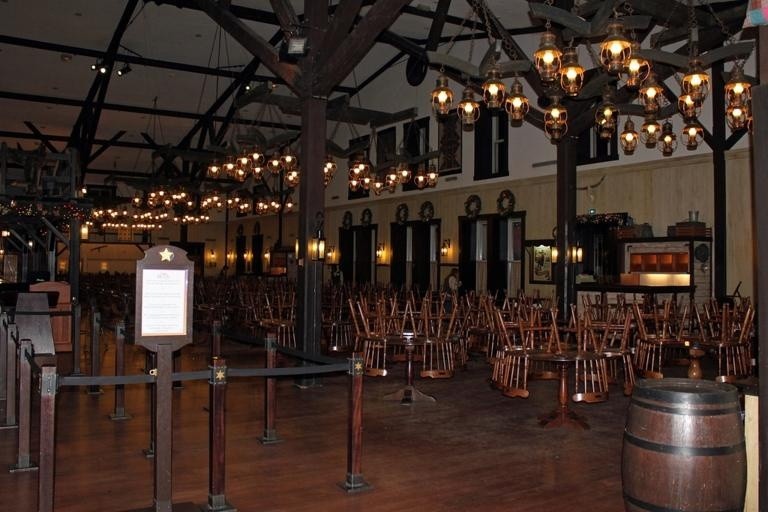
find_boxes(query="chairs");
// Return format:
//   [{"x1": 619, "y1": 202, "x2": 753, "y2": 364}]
[
  {"x1": 344, "y1": 281, "x2": 754, "y2": 424},
  {"x1": 194, "y1": 272, "x2": 344, "y2": 360},
  {"x1": 61, "y1": 270, "x2": 135, "y2": 327}
]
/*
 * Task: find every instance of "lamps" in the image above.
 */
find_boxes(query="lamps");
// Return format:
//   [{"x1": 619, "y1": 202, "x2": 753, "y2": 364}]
[
  {"x1": 726, "y1": 101, "x2": 749, "y2": 131},
  {"x1": 533, "y1": 19, "x2": 563, "y2": 84},
  {"x1": 430, "y1": 73, "x2": 454, "y2": 123},
  {"x1": 504, "y1": 77, "x2": 531, "y2": 128},
  {"x1": 726, "y1": 61, "x2": 751, "y2": 107},
  {"x1": 594, "y1": 88, "x2": 621, "y2": 135},
  {"x1": 542, "y1": 96, "x2": 569, "y2": 144},
  {"x1": 681, "y1": 122, "x2": 704, "y2": 151},
  {"x1": 558, "y1": 41, "x2": 585, "y2": 97},
  {"x1": 639, "y1": 74, "x2": 663, "y2": 114},
  {"x1": 619, "y1": 108, "x2": 640, "y2": 156},
  {"x1": 619, "y1": 33, "x2": 650, "y2": 89},
  {"x1": 599, "y1": 7, "x2": 632, "y2": 74},
  {"x1": 682, "y1": 22, "x2": 711, "y2": 106},
  {"x1": 482, "y1": 61, "x2": 506, "y2": 114},
  {"x1": 78, "y1": 49, "x2": 440, "y2": 271},
  {"x1": 677, "y1": 91, "x2": 702, "y2": 121},
  {"x1": 658, "y1": 118, "x2": 678, "y2": 156},
  {"x1": 456, "y1": 86, "x2": 481, "y2": 132},
  {"x1": 639, "y1": 112, "x2": 661, "y2": 149}
]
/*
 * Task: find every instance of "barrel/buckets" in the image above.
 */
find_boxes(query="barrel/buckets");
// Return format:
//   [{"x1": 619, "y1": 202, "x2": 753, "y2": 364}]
[{"x1": 620, "y1": 377, "x2": 748, "y2": 512}]
